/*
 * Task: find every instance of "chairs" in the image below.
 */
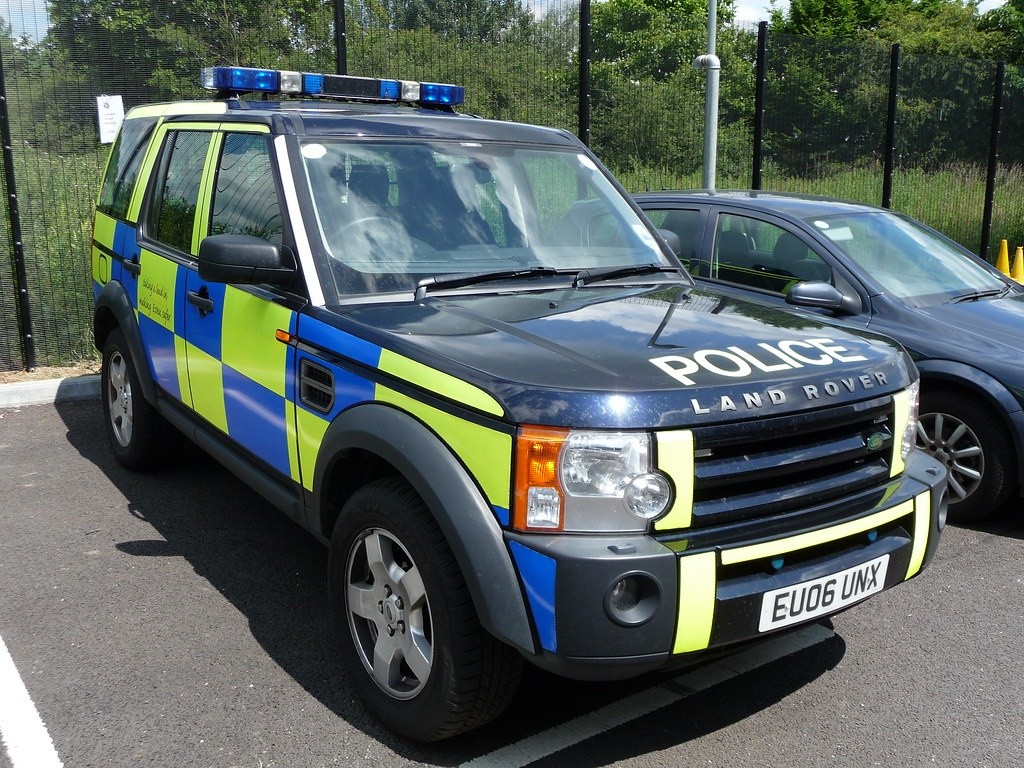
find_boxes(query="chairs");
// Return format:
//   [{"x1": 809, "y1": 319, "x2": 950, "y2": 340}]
[
  {"x1": 405, "y1": 170, "x2": 497, "y2": 253},
  {"x1": 323, "y1": 165, "x2": 412, "y2": 238},
  {"x1": 770, "y1": 235, "x2": 826, "y2": 283},
  {"x1": 718, "y1": 230, "x2": 759, "y2": 285}
]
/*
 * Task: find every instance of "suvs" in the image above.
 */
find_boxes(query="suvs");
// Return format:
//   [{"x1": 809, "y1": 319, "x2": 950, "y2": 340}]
[{"x1": 87, "y1": 64, "x2": 953, "y2": 745}]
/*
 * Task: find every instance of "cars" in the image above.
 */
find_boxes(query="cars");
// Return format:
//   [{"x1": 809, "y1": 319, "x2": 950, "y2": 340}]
[{"x1": 549, "y1": 186, "x2": 1024, "y2": 529}]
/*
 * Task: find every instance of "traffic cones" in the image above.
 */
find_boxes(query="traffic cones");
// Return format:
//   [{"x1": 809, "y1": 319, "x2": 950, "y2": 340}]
[
  {"x1": 1010, "y1": 246, "x2": 1024, "y2": 286},
  {"x1": 994, "y1": 237, "x2": 1012, "y2": 279}
]
